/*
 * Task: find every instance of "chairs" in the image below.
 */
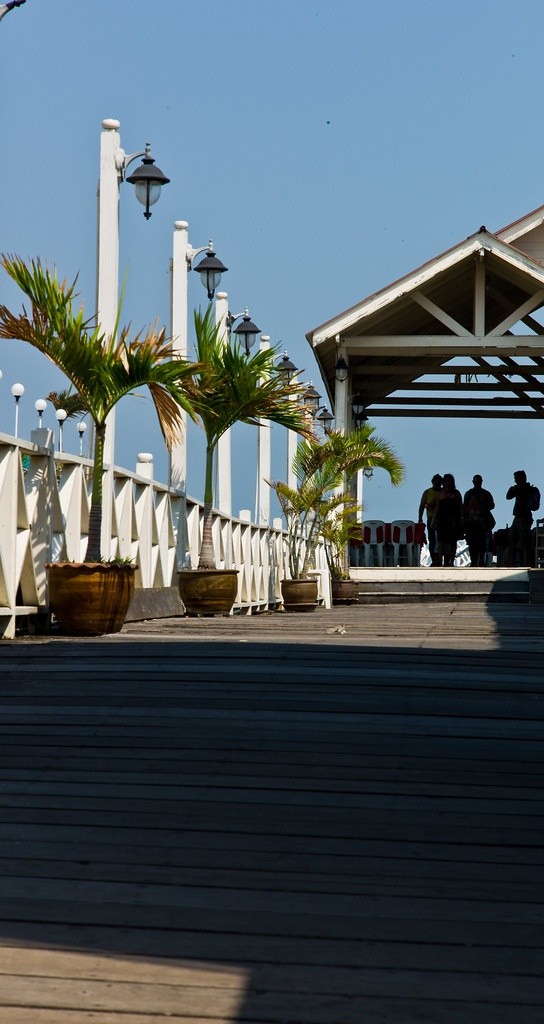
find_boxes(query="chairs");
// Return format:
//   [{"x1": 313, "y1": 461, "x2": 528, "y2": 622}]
[
  {"x1": 360, "y1": 520, "x2": 388, "y2": 567},
  {"x1": 385, "y1": 519, "x2": 415, "y2": 567}
]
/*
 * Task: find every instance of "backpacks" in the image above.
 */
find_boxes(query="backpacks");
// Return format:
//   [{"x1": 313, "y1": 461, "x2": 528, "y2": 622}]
[{"x1": 524, "y1": 487, "x2": 540, "y2": 511}]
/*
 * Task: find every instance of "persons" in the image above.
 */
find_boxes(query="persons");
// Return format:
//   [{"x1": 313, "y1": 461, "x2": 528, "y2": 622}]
[
  {"x1": 461, "y1": 475, "x2": 495, "y2": 567},
  {"x1": 500, "y1": 471, "x2": 540, "y2": 568},
  {"x1": 418, "y1": 475, "x2": 462, "y2": 567}
]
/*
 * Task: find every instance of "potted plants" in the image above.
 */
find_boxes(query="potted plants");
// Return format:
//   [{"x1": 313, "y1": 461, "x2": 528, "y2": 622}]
[
  {"x1": 316, "y1": 491, "x2": 368, "y2": 602},
  {"x1": 263, "y1": 418, "x2": 408, "y2": 613},
  {"x1": 150, "y1": 303, "x2": 318, "y2": 618},
  {"x1": 0, "y1": 251, "x2": 219, "y2": 637}
]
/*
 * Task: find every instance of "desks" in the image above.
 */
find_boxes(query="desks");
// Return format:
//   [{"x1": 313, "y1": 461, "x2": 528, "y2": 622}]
[{"x1": 353, "y1": 522, "x2": 427, "y2": 567}]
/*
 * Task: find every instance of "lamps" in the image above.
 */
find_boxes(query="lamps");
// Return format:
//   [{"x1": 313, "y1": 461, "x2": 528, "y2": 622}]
[
  {"x1": 349, "y1": 391, "x2": 369, "y2": 429},
  {"x1": 333, "y1": 353, "x2": 350, "y2": 384},
  {"x1": 363, "y1": 465, "x2": 374, "y2": 479}
]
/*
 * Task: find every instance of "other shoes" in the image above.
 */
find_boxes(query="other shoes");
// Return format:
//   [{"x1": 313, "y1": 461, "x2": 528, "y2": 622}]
[
  {"x1": 479, "y1": 560, "x2": 486, "y2": 568},
  {"x1": 470, "y1": 561, "x2": 478, "y2": 567},
  {"x1": 429, "y1": 562, "x2": 442, "y2": 567}
]
